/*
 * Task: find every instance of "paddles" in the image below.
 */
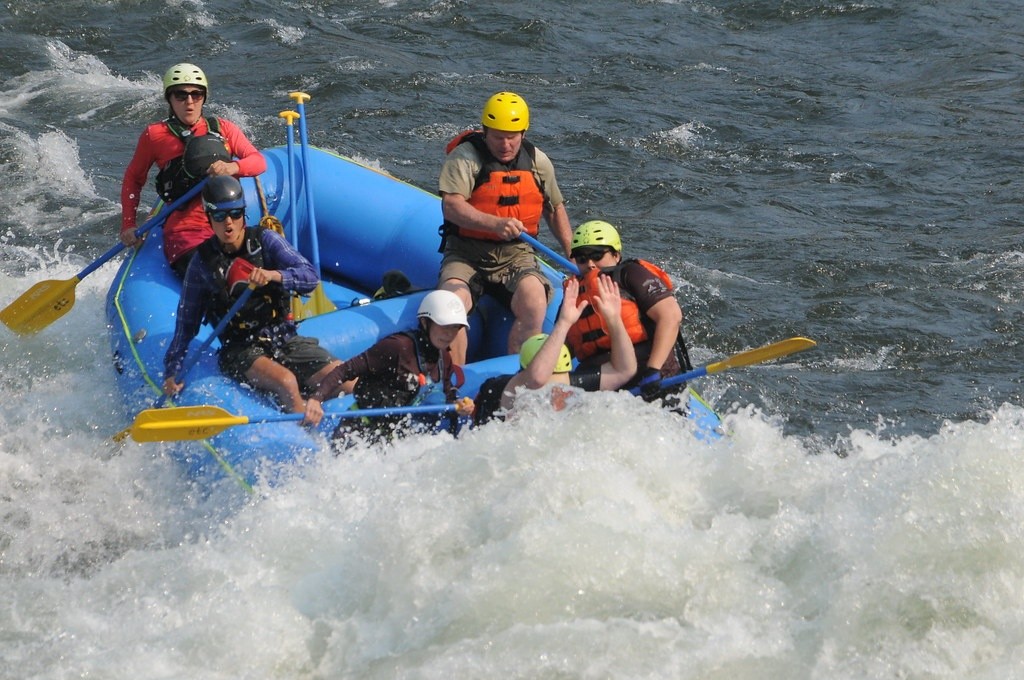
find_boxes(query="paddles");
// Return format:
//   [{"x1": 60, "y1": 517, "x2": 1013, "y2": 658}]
[
  {"x1": 114, "y1": 273, "x2": 270, "y2": 443},
  {"x1": 517, "y1": 229, "x2": 585, "y2": 278},
  {"x1": 0, "y1": 171, "x2": 216, "y2": 335},
  {"x1": 632, "y1": 334, "x2": 819, "y2": 396},
  {"x1": 130, "y1": 395, "x2": 471, "y2": 444},
  {"x1": 276, "y1": 91, "x2": 337, "y2": 320}
]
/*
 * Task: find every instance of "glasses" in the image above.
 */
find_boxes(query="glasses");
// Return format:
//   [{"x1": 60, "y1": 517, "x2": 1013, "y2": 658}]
[
  {"x1": 576, "y1": 248, "x2": 612, "y2": 264},
  {"x1": 209, "y1": 209, "x2": 244, "y2": 222},
  {"x1": 167, "y1": 89, "x2": 205, "y2": 102}
]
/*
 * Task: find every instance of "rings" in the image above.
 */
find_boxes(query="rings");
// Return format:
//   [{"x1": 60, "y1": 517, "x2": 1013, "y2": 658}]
[{"x1": 164, "y1": 386, "x2": 167, "y2": 389}]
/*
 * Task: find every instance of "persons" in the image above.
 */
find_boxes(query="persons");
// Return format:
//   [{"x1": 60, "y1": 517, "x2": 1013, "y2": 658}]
[
  {"x1": 162, "y1": 174, "x2": 358, "y2": 414},
  {"x1": 300, "y1": 291, "x2": 474, "y2": 456},
  {"x1": 554, "y1": 222, "x2": 687, "y2": 400},
  {"x1": 438, "y1": 91, "x2": 578, "y2": 368},
  {"x1": 497, "y1": 275, "x2": 638, "y2": 410},
  {"x1": 119, "y1": 63, "x2": 267, "y2": 275}
]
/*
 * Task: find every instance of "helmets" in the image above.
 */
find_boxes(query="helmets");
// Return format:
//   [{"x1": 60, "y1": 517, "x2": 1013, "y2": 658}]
[
  {"x1": 202, "y1": 176, "x2": 246, "y2": 214},
  {"x1": 518, "y1": 333, "x2": 571, "y2": 373},
  {"x1": 482, "y1": 92, "x2": 529, "y2": 132},
  {"x1": 571, "y1": 220, "x2": 621, "y2": 254},
  {"x1": 164, "y1": 63, "x2": 209, "y2": 100},
  {"x1": 416, "y1": 290, "x2": 472, "y2": 331}
]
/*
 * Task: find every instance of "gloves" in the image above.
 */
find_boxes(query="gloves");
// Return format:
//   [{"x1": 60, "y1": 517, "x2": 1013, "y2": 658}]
[{"x1": 638, "y1": 368, "x2": 662, "y2": 397}]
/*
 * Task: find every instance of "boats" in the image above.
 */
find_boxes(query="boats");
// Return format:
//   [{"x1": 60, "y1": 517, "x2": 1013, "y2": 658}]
[{"x1": 103, "y1": 143, "x2": 729, "y2": 521}]
